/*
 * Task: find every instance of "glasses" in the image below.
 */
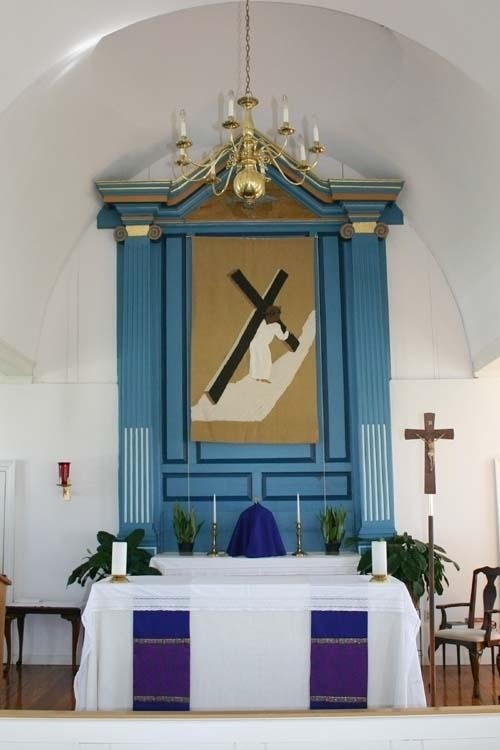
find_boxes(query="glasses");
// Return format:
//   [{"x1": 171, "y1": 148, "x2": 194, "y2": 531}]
[
  {"x1": 171, "y1": 2, "x2": 325, "y2": 200},
  {"x1": 57, "y1": 462, "x2": 71, "y2": 501}
]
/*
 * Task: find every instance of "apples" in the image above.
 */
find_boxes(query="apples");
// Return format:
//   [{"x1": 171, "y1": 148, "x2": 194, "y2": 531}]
[
  {"x1": 371, "y1": 541, "x2": 388, "y2": 576},
  {"x1": 212, "y1": 493, "x2": 216, "y2": 524},
  {"x1": 111, "y1": 541, "x2": 127, "y2": 576},
  {"x1": 296, "y1": 493, "x2": 300, "y2": 523}
]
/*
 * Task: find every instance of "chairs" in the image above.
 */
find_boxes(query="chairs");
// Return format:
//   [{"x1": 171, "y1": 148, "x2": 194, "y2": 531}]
[{"x1": 428, "y1": 568, "x2": 500, "y2": 705}]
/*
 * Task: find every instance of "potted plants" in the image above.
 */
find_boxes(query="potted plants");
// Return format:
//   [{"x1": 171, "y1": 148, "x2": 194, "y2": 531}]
[
  {"x1": 316, "y1": 503, "x2": 348, "y2": 556},
  {"x1": 171, "y1": 503, "x2": 205, "y2": 556},
  {"x1": 358, "y1": 531, "x2": 460, "y2": 609}
]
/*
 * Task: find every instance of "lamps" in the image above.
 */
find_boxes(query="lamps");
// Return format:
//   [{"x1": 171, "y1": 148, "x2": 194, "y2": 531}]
[
  {"x1": 428, "y1": 568, "x2": 500, "y2": 705},
  {"x1": 171, "y1": 2, "x2": 325, "y2": 200},
  {"x1": 57, "y1": 462, "x2": 71, "y2": 501}
]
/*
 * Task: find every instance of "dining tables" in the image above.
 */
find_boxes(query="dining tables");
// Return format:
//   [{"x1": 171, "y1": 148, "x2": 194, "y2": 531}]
[
  {"x1": 171, "y1": 503, "x2": 205, "y2": 556},
  {"x1": 358, "y1": 531, "x2": 460, "y2": 609},
  {"x1": 316, "y1": 503, "x2": 348, "y2": 556}
]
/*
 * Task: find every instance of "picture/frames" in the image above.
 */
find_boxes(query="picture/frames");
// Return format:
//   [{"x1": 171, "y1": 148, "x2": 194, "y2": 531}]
[{"x1": 4, "y1": 603, "x2": 84, "y2": 675}]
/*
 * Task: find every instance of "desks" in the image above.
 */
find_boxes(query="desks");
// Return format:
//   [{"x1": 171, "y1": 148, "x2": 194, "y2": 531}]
[
  {"x1": 4, "y1": 603, "x2": 84, "y2": 675},
  {"x1": 69, "y1": 552, "x2": 426, "y2": 709}
]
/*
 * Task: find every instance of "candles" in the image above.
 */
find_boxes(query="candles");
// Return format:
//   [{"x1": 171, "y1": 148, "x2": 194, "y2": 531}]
[
  {"x1": 111, "y1": 541, "x2": 127, "y2": 576},
  {"x1": 296, "y1": 493, "x2": 300, "y2": 523},
  {"x1": 371, "y1": 541, "x2": 388, "y2": 576},
  {"x1": 212, "y1": 493, "x2": 216, "y2": 524}
]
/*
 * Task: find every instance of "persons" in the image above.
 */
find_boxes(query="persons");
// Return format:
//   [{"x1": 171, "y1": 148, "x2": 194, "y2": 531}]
[{"x1": 415, "y1": 433, "x2": 446, "y2": 470}]
